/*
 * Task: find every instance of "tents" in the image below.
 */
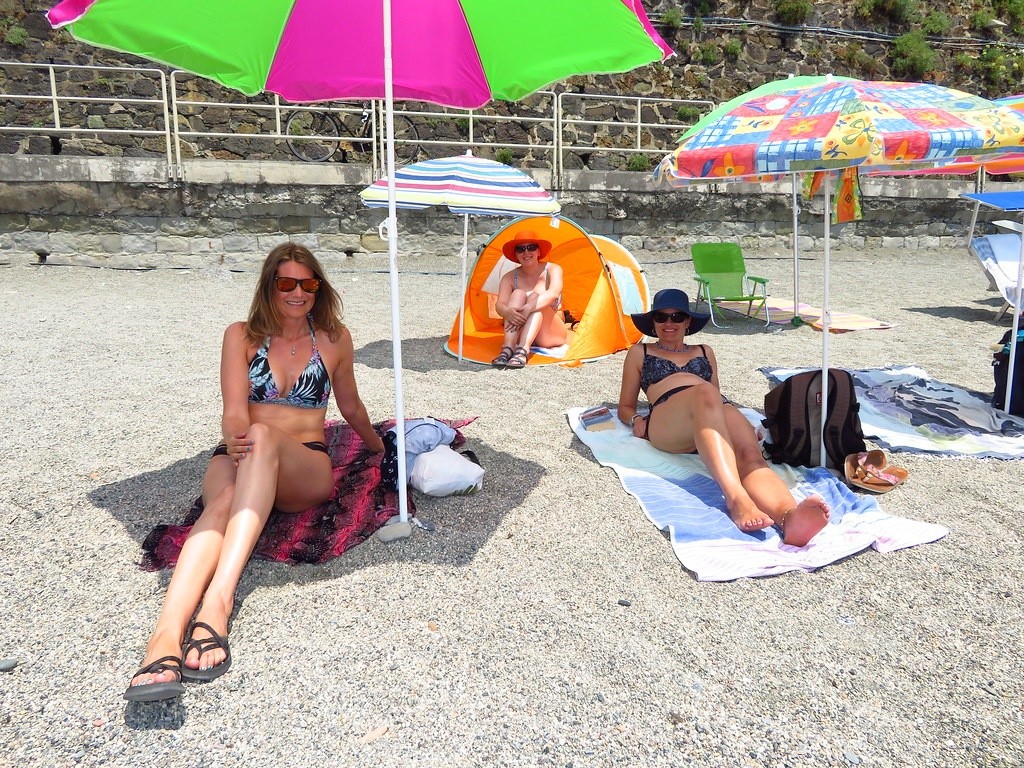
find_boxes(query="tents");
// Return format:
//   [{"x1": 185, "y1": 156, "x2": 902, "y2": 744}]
[{"x1": 441, "y1": 215, "x2": 653, "y2": 371}]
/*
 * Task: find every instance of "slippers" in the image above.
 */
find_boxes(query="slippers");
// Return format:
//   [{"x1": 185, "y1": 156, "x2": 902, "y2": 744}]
[
  {"x1": 123, "y1": 655, "x2": 185, "y2": 702},
  {"x1": 181, "y1": 622, "x2": 232, "y2": 681},
  {"x1": 852, "y1": 466, "x2": 909, "y2": 493},
  {"x1": 492, "y1": 346, "x2": 514, "y2": 366},
  {"x1": 506, "y1": 347, "x2": 529, "y2": 367},
  {"x1": 843, "y1": 450, "x2": 887, "y2": 488}
]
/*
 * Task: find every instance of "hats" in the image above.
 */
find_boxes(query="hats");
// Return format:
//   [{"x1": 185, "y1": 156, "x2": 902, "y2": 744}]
[
  {"x1": 630, "y1": 288, "x2": 711, "y2": 338},
  {"x1": 503, "y1": 230, "x2": 552, "y2": 264}
]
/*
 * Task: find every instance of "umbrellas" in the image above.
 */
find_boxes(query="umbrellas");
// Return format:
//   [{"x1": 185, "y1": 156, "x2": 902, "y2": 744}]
[
  {"x1": 358, "y1": 145, "x2": 560, "y2": 358},
  {"x1": 652, "y1": 73, "x2": 1024, "y2": 471},
  {"x1": 42, "y1": 0, "x2": 673, "y2": 530}
]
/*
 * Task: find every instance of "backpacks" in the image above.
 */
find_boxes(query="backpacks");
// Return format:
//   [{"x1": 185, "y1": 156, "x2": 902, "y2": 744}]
[
  {"x1": 994, "y1": 323, "x2": 1024, "y2": 416},
  {"x1": 761, "y1": 368, "x2": 867, "y2": 468}
]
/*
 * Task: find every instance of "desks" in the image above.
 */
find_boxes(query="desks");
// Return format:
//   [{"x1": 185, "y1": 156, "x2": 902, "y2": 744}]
[{"x1": 957, "y1": 191, "x2": 1023, "y2": 246}]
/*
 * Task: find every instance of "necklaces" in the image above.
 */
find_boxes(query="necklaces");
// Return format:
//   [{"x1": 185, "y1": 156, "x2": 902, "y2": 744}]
[
  {"x1": 279, "y1": 334, "x2": 307, "y2": 355},
  {"x1": 655, "y1": 340, "x2": 692, "y2": 352}
]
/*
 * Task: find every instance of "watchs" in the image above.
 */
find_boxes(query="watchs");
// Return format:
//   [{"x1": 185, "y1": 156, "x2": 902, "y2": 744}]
[{"x1": 629, "y1": 414, "x2": 642, "y2": 426}]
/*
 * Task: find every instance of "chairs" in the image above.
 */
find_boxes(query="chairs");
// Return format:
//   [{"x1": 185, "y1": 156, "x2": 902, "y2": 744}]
[
  {"x1": 969, "y1": 233, "x2": 1024, "y2": 323},
  {"x1": 692, "y1": 241, "x2": 771, "y2": 329}
]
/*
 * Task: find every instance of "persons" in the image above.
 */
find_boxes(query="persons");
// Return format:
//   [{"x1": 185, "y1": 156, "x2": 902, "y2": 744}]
[
  {"x1": 616, "y1": 289, "x2": 829, "y2": 547},
  {"x1": 119, "y1": 242, "x2": 385, "y2": 700},
  {"x1": 489, "y1": 230, "x2": 566, "y2": 368}
]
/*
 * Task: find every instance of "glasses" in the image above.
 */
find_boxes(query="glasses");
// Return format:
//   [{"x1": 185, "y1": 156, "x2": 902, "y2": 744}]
[
  {"x1": 515, "y1": 244, "x2": 538, "y2": 253},
  {"x1": 653, "y1": 312, "x2": 688, "y2": 323},
  {"x1": 273, "y1": 277, "x2": 322, "y2": 293}
]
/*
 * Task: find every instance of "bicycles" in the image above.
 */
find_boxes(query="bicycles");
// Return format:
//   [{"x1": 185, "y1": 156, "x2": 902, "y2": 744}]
[{"x1": 286, "y1": 99, "x2": 422, "y2": 165}]
